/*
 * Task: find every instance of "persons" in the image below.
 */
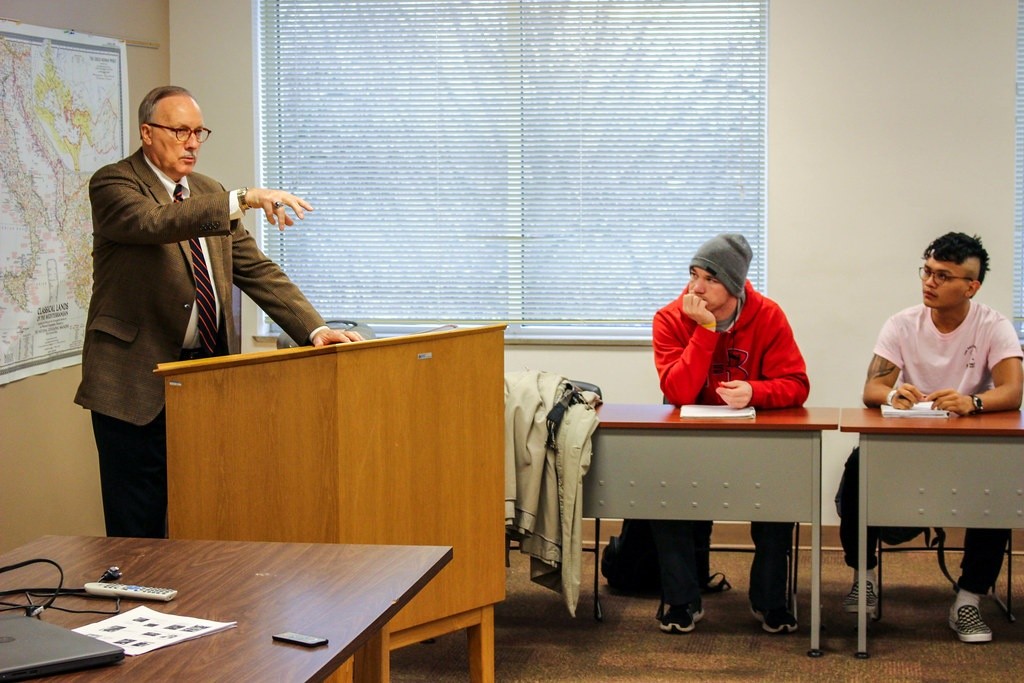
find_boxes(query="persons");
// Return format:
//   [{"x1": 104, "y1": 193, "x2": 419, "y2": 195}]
[
  {"x1": 653, "y1": 232, "x2": 810, "y2": 633},
  {"x1": 73, "y1": 85, "x2": 367, "y2": 539},
  {"x1": 834, "y1": 232, "x2": 1024, "y2": 642}
]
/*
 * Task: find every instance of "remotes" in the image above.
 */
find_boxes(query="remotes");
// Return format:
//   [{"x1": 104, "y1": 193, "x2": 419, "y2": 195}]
[{"x1": 84, "y1": 582, "x2": 177, "y2": 601}]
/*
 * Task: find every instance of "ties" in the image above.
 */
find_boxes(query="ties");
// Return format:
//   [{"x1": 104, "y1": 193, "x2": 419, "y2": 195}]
[{"x1": 173, "y1": 184, "x2": 218, "y2": 358}]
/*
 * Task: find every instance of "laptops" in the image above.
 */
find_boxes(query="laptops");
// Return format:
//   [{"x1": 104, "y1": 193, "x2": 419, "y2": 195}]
[{"x1": 0, "y1": 613, "x2": 126, "y2": 681}]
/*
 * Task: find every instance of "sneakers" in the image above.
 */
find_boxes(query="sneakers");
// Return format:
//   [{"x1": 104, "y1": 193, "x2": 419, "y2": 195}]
[
  {"x1": 748, "y1": 598, "x2": 799, "y2": 634},
  {"x1": 841, "y1": 579, "x2": 881, "y2": 613},
  {"x1": 949, "y1": 604, "x2": 992, "y2": 643},
  {"x1": 658, "y1": 599, "x2": 705, "y2": 634}
]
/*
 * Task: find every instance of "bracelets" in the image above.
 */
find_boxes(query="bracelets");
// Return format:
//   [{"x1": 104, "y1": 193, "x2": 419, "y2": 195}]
[
  {"x1": 886, "y1": 390, "x2": 897, "y2": 405},
  {"x1": 703, "y1": 323, "x2": 716, "y2": 328}
]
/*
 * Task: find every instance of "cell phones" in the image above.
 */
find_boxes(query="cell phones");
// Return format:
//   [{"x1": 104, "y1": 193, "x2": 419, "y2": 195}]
[{"x1": 272, "y1": 633, "x2": 329, "y2": 648}]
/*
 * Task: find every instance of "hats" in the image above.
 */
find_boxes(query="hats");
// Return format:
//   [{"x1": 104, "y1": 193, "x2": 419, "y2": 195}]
[{"x1": 688, "y1": 231, "x2": 753, "y2": 298}]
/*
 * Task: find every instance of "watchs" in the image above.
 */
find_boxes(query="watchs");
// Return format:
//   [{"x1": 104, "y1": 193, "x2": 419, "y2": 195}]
[
  {"x1": 237, "y1": 187, "x2": 249, "y2": 210},
  {"x1": 969, "y1": 395, "x2": 983, "y2": 415}
]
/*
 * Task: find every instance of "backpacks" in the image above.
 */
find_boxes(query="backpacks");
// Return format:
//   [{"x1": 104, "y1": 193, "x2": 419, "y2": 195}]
[{"x1": 602, "y1": 518, "x2": 731, "y2": 601}]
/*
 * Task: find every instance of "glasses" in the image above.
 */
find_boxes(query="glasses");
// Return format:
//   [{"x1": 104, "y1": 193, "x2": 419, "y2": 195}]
[
  {"x1": 140, "y1": 122, "x2": 213, "y2": 143},
  {"x1": 919, "y1": 267, "x2": 974, "y2": 285}
]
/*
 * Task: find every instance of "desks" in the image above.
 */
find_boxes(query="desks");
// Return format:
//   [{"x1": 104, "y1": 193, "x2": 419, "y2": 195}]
[
  {"x1": 0, "y1": 535, "x2": 453, "y2": 683},
  {"x1": 583, "y1": 405, "x2": 839, "y2": 657},
  {"x1": 839, "y1": 407, "x2": 1024, "y2": 659}
]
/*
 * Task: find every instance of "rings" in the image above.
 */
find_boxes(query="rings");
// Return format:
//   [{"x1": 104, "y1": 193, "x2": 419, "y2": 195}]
[{"x1": 274, "y1": 201, "x2": 283, "y2": 208}]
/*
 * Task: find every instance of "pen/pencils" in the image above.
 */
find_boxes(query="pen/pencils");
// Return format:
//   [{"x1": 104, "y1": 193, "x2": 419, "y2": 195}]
[
  {"x1": 718, "y1": 381, "x2": 723, "y2": 387},
  {"x1": 899, "y1": 395, "x2": 937, "y2": 401}
]
